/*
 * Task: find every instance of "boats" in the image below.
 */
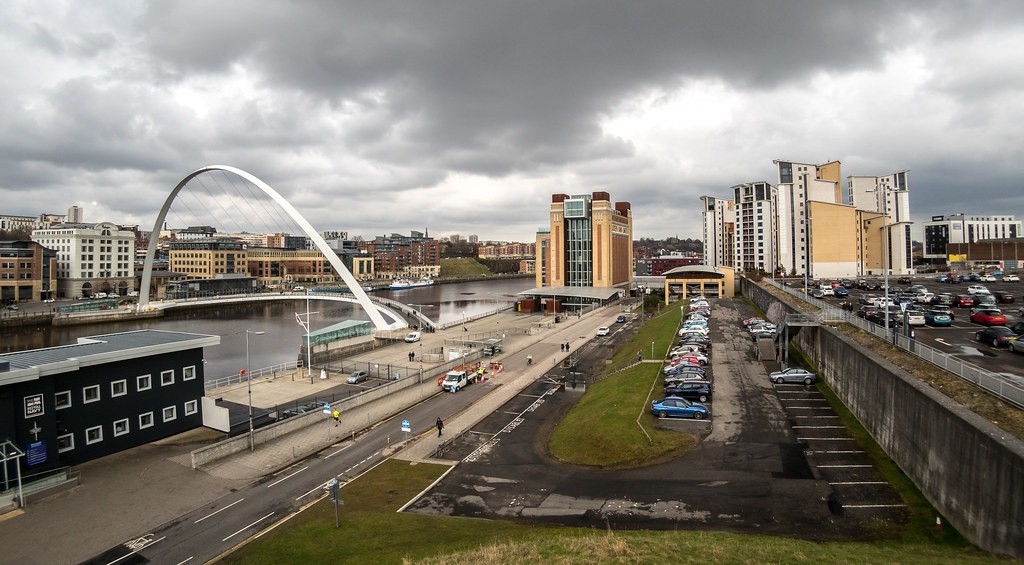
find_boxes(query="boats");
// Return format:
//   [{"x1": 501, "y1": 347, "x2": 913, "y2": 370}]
[{"x1": 389, "y1": 277, "x2": 435, "y2": 290}]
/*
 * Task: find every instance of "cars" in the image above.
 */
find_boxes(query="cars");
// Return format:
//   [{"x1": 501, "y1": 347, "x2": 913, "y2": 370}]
[
  {"x1": 108, "y1": 293, "x2": 120, "y2": 298},
  {"x1": 651, "y1": 295, "x2": 711, "y2": 421},
  {"x1": 596, "y1": 327, "x2": 610, "y2": 336},
  {"x1": 932, "y1": 270, "x2": 1021, "y2": 283},
  {"x1": 44, "y1": 298, "x2": 56, "y2": 303},
  {"x1": 282, "y1": 401, "x2": 325, "y2": 418},
  {"x1": 616, "y1": 316, "x2": 626, "y2": 323},
  {"x1": 347, "y1": 370, "x2": 368, "y2": 384},
  {"x1": 6, "y1": 304, "x2": 19, "y2": 310},
  {"x1": 769, "y1": 367, "x2": 817, "y2": 385},
  {"x1": 742, "y1": 316, "x2": 781, "y2": 339},
  {"x1": 841, "y1": 285, "x2": 1023, "y2": 354},
  {"x1": 808, "y1": 278, "x2": 890, "y2": 300}
]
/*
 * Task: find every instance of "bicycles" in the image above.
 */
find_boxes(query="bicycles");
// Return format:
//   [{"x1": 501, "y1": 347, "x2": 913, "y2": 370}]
[{"x1": 527, "y1": 359, "x2": 531, "y2": 365}]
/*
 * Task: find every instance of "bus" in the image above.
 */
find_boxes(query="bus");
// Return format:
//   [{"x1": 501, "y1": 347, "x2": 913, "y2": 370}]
[{"x1": 481, "y1": 338, "x2": 503, "y2": 356}]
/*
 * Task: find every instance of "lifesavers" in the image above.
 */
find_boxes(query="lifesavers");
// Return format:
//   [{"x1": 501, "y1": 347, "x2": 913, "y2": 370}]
[{"x1": 240, "y1": 369, "x2": 245, "y2": 375}]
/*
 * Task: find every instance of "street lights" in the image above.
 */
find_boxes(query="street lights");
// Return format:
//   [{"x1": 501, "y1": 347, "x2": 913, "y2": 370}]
[
  {"x1": 865, "y1": 181, "x2": 902, "y2": 337},
  {"x1": 245, "y1": 329, "x2": 266, "y2": 451},
  {"x1": 295, "y1": 288, "x2": 320, "y2": 376},
  {"x1": 407, "y1": 303, "x2": 434, "y2": 333},
  {"x1": 462, "y1": 310, "x2": 465, "y2": 348}
]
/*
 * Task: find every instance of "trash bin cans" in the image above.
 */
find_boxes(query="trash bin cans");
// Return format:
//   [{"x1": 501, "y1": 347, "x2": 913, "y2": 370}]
[{"x1": 555, "y1": 316, "x2": 560, "y2": 323}]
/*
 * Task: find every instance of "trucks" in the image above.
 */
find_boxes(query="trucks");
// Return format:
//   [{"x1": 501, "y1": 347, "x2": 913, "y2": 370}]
[
  {"x1": 127, "y1": 291, "x2": 140, "y2": 297},
  {"x1": 95, "y1": 292, "x2": 108, "y2": 299},
  {"x1": 441, "y1": 368, "x2": 478, "y2": 392},
  {"x1": 293, "y1": 286, "x2": 304, "y2": 292},
  {"x1": 404, "y1": 332, "x2": 420, "y2": 343}
]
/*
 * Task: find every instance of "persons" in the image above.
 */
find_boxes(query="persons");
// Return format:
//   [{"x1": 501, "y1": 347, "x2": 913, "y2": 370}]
[
  {"x1": 566, "y1": 342, "x2": 570, "y2": 352},
  {"x1": 435, "y1": 417, "x2": 444, "y2": 438},
  {"x1": 412, "y1": 351, "x2": 415, "y2": 361},
  {"x1": 408, "y1": 352, "x2": 412, "y2": 362},
  {"x1": 526, "y1": 353, "x2": 532, "y2": 365},
  {"x1": 413, "y1": 324, "x2": 418, "y2": 330},
  {"x1": 561, "y1": 344, "x2": 564, "y2": 352},
  {"x1": 429, "y1": 324, "x2": 435, "y2": 333},
  {"x1": 332, "y1": 408, "x2": 341, "y2": 427},
  {"x1": 477, "y1": 369, "x2": 483, "y2": 380},
  {"x1": 638, "y1": 349, "x2": 644, "y2": 361}
]
